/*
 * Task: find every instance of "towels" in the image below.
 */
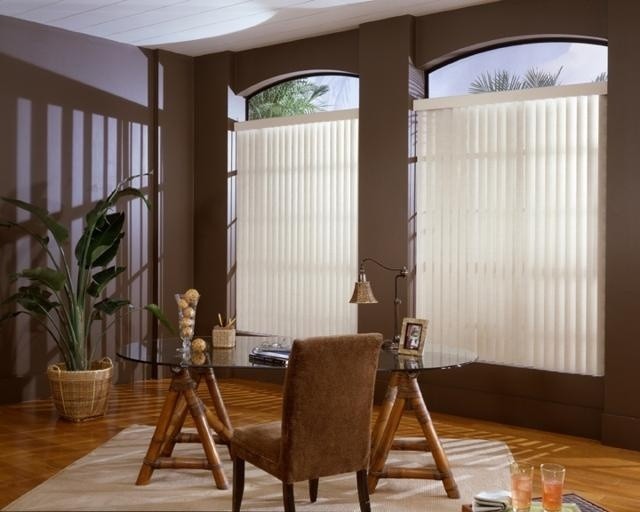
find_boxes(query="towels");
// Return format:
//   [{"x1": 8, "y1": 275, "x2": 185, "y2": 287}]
[{"x1": 472, "y1": 491, "x2": 513, "y2": 511}]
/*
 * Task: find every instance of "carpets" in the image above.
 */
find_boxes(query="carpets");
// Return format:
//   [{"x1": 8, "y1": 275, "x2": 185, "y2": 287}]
[{"x1": 2, "y1": 412, "x2": 519, "y2": 512}]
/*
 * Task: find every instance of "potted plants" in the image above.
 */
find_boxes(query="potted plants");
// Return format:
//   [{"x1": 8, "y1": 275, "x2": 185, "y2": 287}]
[{"x1": 0, "y1": 166, "x2": 178, "y2": 421}]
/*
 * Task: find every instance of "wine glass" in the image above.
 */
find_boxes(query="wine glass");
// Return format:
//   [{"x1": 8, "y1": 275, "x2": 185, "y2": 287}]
[{"x1": 174, "y1": 292, "x2": 201, "y2": 354}]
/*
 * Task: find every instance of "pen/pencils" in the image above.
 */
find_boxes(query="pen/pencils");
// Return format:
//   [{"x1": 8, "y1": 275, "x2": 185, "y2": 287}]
[
  {"x1": 218, "y1": 313, "x2": 236, "y2": 327},
  {"x1": 262, "y1": 349, "x2": 291, "y2": 353}
]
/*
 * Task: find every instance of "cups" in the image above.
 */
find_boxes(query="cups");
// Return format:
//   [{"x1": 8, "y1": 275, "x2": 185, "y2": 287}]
[
  {"x1": 510, "y1": 461, "x2": 533, "y2": 511},
  {"x1": 539, "y1": 462, "x2": 567, "y2": 511}
]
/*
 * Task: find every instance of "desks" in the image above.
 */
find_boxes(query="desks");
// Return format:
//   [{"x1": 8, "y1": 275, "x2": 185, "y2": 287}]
[{"x1": 113, "y1": 334, "x2": 480, "y2": 500}]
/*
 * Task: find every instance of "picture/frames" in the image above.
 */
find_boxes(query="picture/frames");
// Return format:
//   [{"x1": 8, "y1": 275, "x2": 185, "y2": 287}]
[{"x1": 397, "y1": 318, "x2": 429, "y2": 357}]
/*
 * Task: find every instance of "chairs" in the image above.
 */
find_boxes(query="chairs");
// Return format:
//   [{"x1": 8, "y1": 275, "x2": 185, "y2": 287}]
[{"x1": 229, "y1": 331, "x2": 385, "y2": 512}]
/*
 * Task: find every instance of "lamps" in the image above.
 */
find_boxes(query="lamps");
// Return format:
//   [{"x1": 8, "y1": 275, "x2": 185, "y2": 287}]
[{"x1": 351, "y1": 258, "x2": 409, "y2": 349}]
